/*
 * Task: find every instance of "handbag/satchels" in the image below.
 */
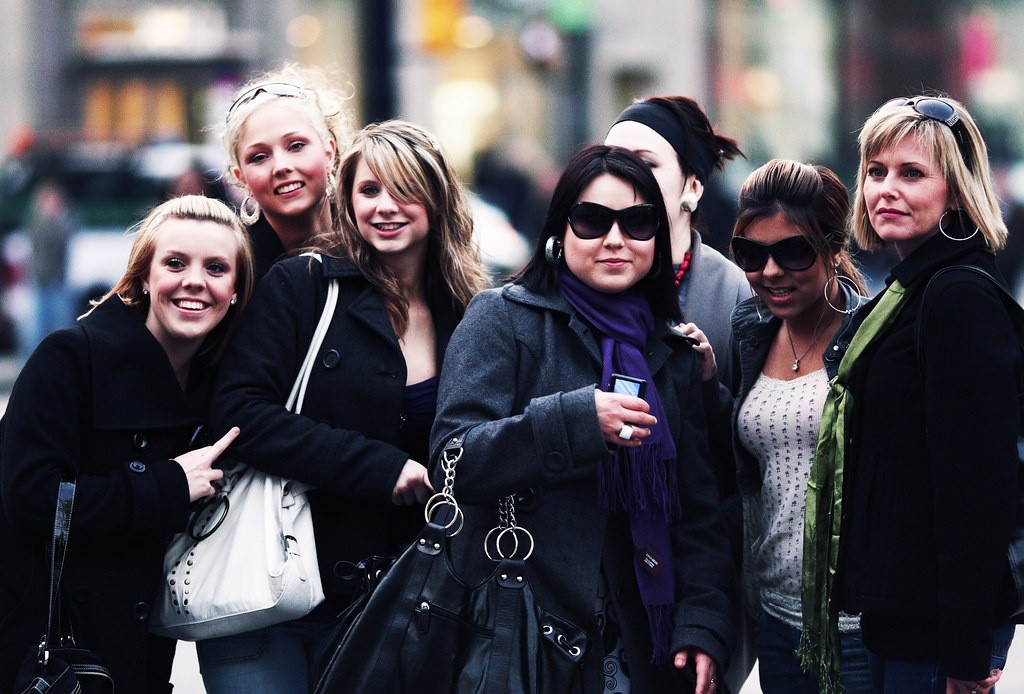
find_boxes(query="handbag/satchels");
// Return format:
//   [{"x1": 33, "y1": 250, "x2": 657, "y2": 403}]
[
  {"x1": 156, "y1": 459, "x2": 326, "y2": 642},
  {"x1": 302, "y1": 426, "x2": 588, "y2": 694},
  {"x1": 13, "y1": 473, "x2": 114, "y2": 694}
]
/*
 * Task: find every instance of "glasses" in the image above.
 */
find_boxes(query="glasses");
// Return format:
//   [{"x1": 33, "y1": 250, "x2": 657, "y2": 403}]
[
  {"x1": 872, "y1": 97, "x2": 965, "y2": 161},
  {"x1": 731, "y1": 233, "x2": 836, "y2": 273},
  {"x1": 226, "y1": 82, "x2": 307, "y2": 122},
  {"x1": 566, "y1": 200, "x2": 662, "y2": 242}
]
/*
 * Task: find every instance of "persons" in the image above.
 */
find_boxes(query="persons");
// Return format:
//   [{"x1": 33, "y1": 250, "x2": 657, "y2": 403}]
[
  {"x1": 470, "y1": 135, "x2": 553, "y2": 252},
  {"x1": 967, "y1": 112, "x2": 1024, "y2": 297},
  {"x1": 428, "y1": 92, "x2": 873, "y2": 694},
  {"x1": 796, "y1": 81, "x2": 1024, "y2": 694},
  {"x1": 0, "y1": 59, "x2": 501, "y2": 694},
  {"x1": 611, "y1": 56, "x2": 739, "y2": 255}
]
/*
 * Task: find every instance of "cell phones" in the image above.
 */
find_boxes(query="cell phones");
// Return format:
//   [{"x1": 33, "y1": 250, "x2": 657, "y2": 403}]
[{"x1": 609, "y1": 374, "x2": 647, "y2": 428}]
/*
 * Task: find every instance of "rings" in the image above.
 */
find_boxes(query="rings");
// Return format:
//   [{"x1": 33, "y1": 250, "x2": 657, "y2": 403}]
[
  {"x1": 971, "y1": 683, "x2": 981, "y2": 694},
  {"x1": 619, "y1": 422, "x2": 634, "y2": 440},
  {"x1": 709, "y1": 679, "x2": 715, "y2": 683}
]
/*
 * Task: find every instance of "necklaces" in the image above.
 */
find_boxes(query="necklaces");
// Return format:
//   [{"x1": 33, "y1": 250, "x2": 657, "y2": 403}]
[
  {"x1": 675, "y1": 251, "x2": 692, "y2": 289},
  {"x1": 786, "y1": 311, "x2": 835, "y2": 372}
]
